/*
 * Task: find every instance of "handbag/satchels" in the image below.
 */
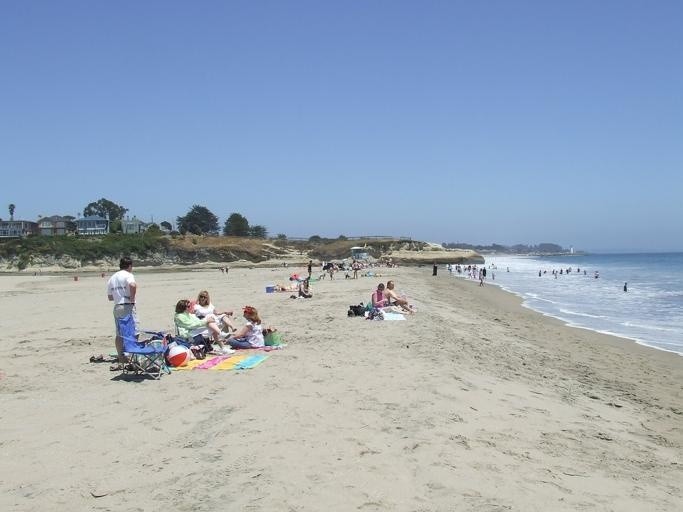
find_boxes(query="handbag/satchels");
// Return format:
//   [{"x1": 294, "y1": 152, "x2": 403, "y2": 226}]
[
  {"x1": 265, "y1": 328, "x2": 279, "y2": 345},
  {"x1": 347, "y1": 305, "x2": 366, "y2": 317}
]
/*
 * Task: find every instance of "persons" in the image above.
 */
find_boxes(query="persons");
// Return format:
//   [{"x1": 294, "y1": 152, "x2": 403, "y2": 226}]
[
  {"x1": 175, "y1": 290, "x2": 237, "y2": 356},
  {"x1": 107, "y1": 257, "x2": 137, "y2": 362},
  {"x1": 624, "y1": 282, "x2": 627, "y2": 291},
  {"x1": 372, "y1": 280, "x2": 417, "y2": 314},
  {"x1": 225, "y1": 306, "x2": 264, "y2": 348},
  {"x1": 539, "y1": 267, "x2": 599, "y2": 278},
  {"x1": 305, "y1": 256, "x2": 399, "y2": 280},
  {"x1": 300, "y1": 280, "x2": 313, "y2": 298},
  {"x1": 448, "y1": 263, "x2": 497, "y2": 287}
]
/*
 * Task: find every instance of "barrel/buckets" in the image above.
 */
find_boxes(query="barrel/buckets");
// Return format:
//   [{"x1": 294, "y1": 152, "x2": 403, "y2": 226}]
[
  {"x1": 265, "y1": 284, "x2": 275, "y2": 292},
  {"x1": 74, "y1": 276, "x2": 78, "y2": 281}
]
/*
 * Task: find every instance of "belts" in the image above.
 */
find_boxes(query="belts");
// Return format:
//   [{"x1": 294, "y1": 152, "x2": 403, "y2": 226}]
[{"x1": 118, "y1": 303, "x2": 134, "y2": 306}]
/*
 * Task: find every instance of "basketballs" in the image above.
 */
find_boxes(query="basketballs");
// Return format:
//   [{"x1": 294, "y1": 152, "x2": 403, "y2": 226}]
[{"x1": 169, "y1": 344, "x2": 191, "y2": 367}]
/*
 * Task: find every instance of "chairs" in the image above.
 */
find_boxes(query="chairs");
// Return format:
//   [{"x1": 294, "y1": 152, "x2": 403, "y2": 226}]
[{"x1": 116, "y1": 314, "x2": 214, "y2": 381}]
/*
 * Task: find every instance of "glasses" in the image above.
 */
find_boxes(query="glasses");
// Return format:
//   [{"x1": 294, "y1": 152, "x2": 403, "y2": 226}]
[
  {"x1": 200, "y1": 296, "x2": 207, "y2": 299},
  {"x1": 187, "y1": 306, "x2": 190, "y2": 308}
]
